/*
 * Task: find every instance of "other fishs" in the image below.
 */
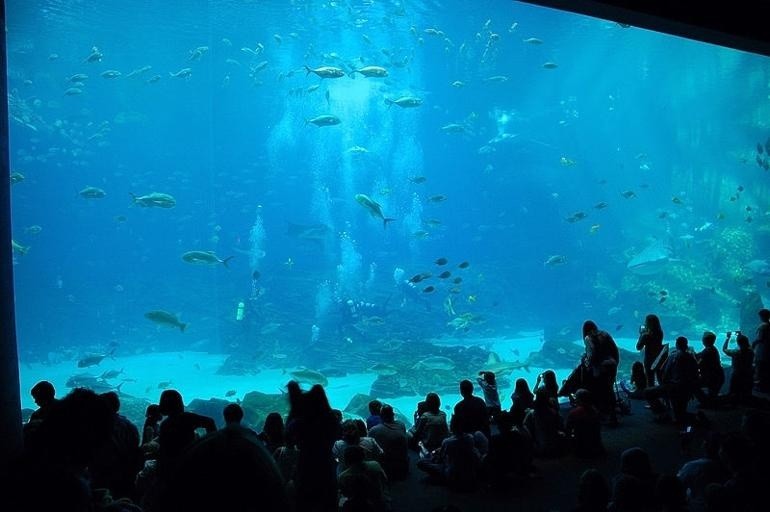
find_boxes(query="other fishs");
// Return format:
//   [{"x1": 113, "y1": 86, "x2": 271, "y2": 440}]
[{"x1": 5, "y1": 0, "x2": 770, "y2": 410}]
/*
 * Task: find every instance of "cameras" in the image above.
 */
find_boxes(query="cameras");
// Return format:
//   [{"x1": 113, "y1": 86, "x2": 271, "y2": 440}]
[
  {"x1": 539, "y1": 374, "x2": 544, "y2": 378},
  {"x1": 641, "y1": 325, "x2": 646, "y2": 330},
  {"x1": 731, "y1": 332, "x2": 737, "y2": 335}
]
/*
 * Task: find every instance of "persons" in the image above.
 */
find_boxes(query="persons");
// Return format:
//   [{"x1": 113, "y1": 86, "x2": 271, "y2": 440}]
[{"x1": 19, "y1": 307, "x2": 770, "y2": 512}]
